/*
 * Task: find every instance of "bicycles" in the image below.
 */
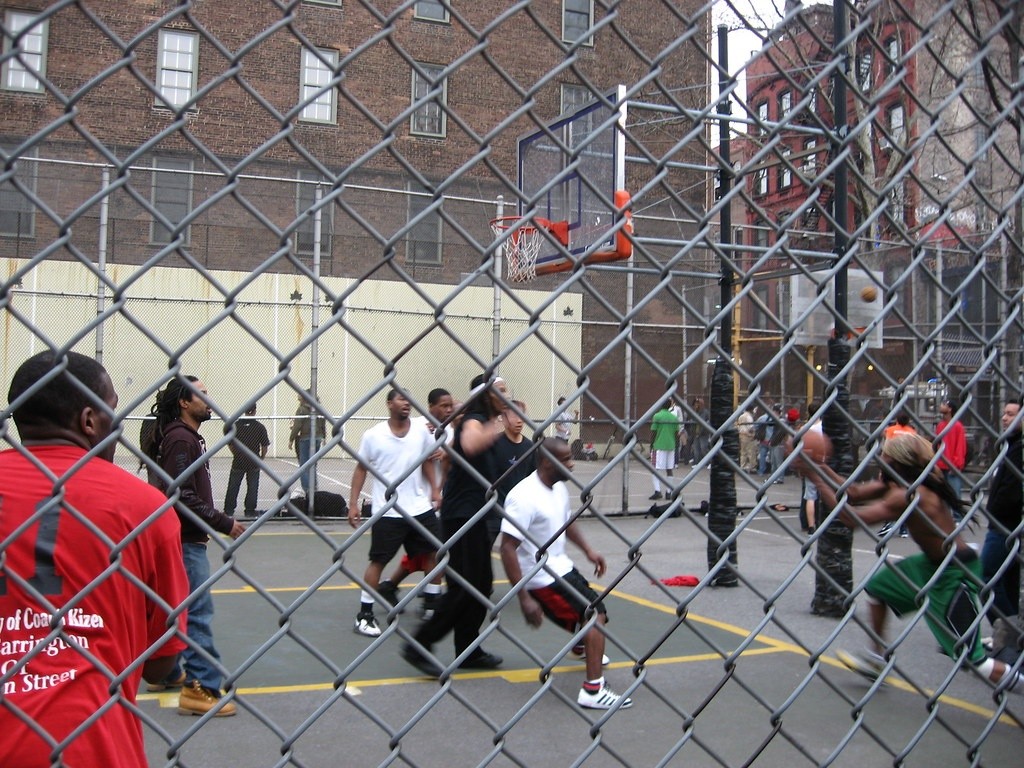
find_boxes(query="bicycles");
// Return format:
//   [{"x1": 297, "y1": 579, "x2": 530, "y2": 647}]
[{"x1": 602, "y1": 417, "x2": 652, "y2": 460}]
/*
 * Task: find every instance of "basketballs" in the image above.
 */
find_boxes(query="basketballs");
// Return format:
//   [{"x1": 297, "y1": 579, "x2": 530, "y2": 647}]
[
  {"x1": 784, "y1": 428, "x2": 834, "y2": 470},
  {"x1": 860, "y1": 286, "x2": 877, "y2": 302}
]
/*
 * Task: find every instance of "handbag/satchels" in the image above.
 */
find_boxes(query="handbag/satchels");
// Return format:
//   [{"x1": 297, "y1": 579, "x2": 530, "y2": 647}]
[{"x1": 754, "y1": 425, "x2": 766, "y2": 440}]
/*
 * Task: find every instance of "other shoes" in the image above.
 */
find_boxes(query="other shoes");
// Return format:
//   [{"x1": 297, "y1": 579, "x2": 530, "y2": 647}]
[
  {"x1": 806, "y1": 527, "x2": 814, "y2": 536},
  {"x1": 692, "y1": 464, "x2": 696, "y2": 468},
  {"x1": 666, "y1": 492, "x2": 671, "y2": 499},
  {"x1": 707, "y1": 464, "x2": 711, "y2": 469},
  {"x1": 649, "y1": 491, "x2": 661, "y2": 499},
  {"x1": 838, "y1": 650, "x2": 884, "y2": 681}
]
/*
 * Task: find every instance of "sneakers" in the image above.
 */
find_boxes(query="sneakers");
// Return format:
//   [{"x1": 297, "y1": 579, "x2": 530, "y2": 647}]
[
  {"x1": 877, "y1": 522, "x2": 893, "y2": 535},
  {"x1": 422, "y1": 604, "x2": 441, "y2": 620},
  {"x1": 565, "y1": 645, "x2": 610, "y2": 665},
  {"x1": 353, "y1": 612, "x2": 380, "y2": 636},
  {"x1": 147, "y1": 673, "x2": 187, "y2": 691},
  {"x1": 577, "y1": 676, "x2": 633, "y2": 709},
  {"x1": 899, "y1": 529, "x2": 908, "y2": 537},
  {"x1": 400, "y1": 645, "x2": 441, "y2": 676},
  {"x1": 177, "y1": 679, "x2": 237, "y2": 716},
  {"x1": 379, "y1": 579, "x2": 404, "y2": 614},
  {"x1": 454, "y1": 647, "x2": 502, "y2": 668}
]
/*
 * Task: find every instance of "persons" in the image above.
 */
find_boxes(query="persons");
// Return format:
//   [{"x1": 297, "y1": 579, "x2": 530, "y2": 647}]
[
  {"x1": 740, "y1": 402, "x2": 825, "y2": 534},
  {"x1": 223, "y1": 401, "x2": 271, "y2": 517},
  {"x1": 978, "y1": 397, "x2": 1024, "y2": 652},
  {"x1": 348, "y1": 386, "x2": 443, "y2": 636},
  {"x1": 789, "y1": 429, "x2": 1024, "y2": 698},
  {"x1": 648, "y1": 394, "x2": 688, "y2": 500},
  {"x1": 376, "y1": 370, "x2": 539, "y2": 677},
  {"x1": 142, "y1": 372, "x2": 247, "y2": 716},
  {"x1": 882, "y1": 412, "x2": 918, "y2": 440},
  {"x1": 288, "y1": 388, "x2": 326, "y2": 496},
  {"x1": 500, "y1": 438, "x2": 633, "y2": 710},
  {"x1": 0, "y1": 348, "x2": 191, "y2": 768},
  {"x1": 554, "y1": 398, "x2": 579, "y2": 444},
  {"x1": 859, "y1": 390, "x2": 892, "y2": 435},
  {"x1": 935, "y1": 401, "x2": 967, "y2": 521},
  {"x1": 683, "y1": 397, "x2": 710, "y2": 470}
]
